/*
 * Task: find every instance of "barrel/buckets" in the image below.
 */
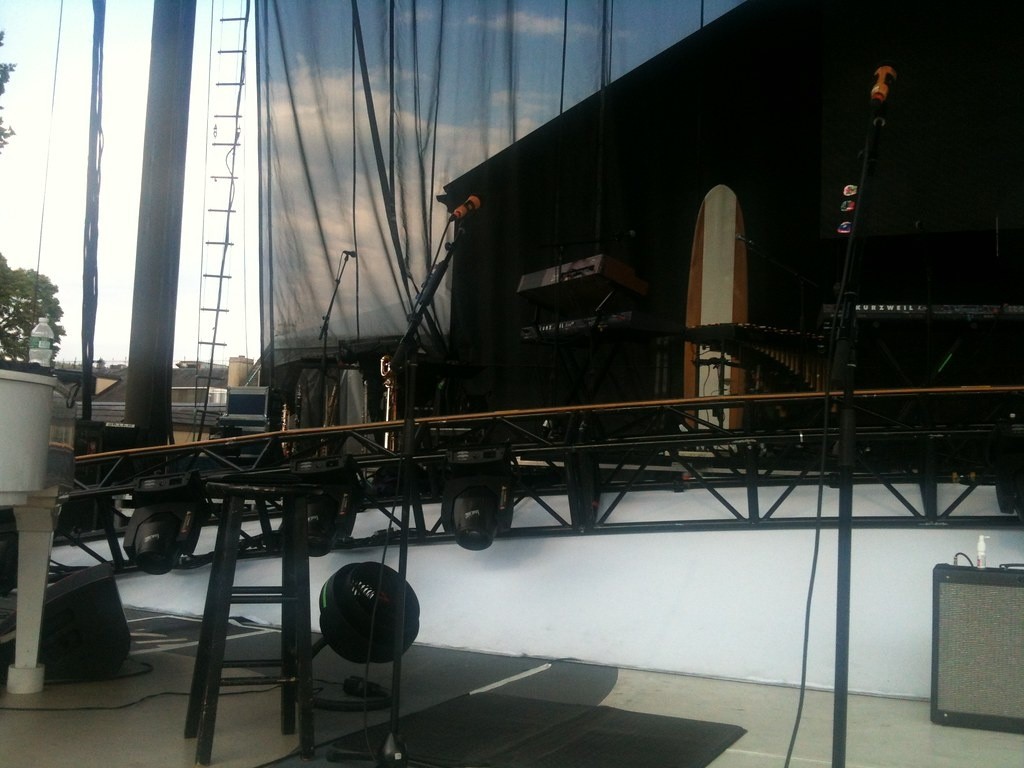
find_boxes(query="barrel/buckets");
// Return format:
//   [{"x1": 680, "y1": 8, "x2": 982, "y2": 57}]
[{"x1": 209, "y1": 427, "x2": 242, "y2": 457}]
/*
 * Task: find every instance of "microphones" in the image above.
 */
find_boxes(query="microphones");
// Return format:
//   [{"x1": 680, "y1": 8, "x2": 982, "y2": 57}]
[
  {"x1": 343, "y1": 251, "x2": 357, "y2": 257},
  {"x1": 448, "y1": 193, "x2": 484, "y2": 222},
  {"x1": 869, "y1": 58, "x2": 899, "y2": 111}
]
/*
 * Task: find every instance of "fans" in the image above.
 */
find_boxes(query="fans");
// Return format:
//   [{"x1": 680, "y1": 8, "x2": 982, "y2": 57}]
[{"x1": 306, "y1": 561, "x2": 421, "y2": 710}]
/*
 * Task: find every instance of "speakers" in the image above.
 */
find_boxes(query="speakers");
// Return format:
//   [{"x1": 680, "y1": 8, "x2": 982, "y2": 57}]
[
  {"x1": 0, "y1": 562, "x2": 131, "y2": 680},
  {"x1": 929, "y1": 564, "x2": 1023, "y2": 736}
]
